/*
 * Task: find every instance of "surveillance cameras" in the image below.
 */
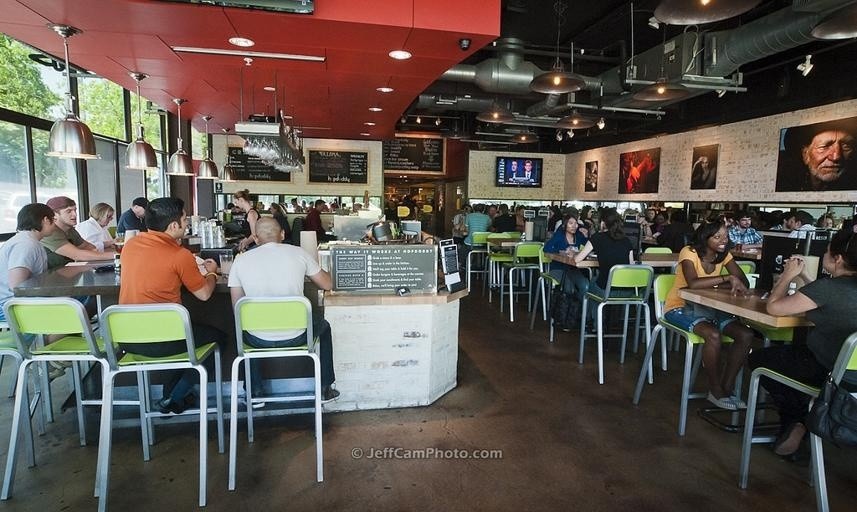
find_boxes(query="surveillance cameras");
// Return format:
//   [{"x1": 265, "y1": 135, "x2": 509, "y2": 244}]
[{"x1": 459, "y1": 39, "x2": 472, "y2": 51}]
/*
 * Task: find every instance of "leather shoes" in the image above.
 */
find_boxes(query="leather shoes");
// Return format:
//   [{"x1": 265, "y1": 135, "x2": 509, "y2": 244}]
[
  {"x1": 708, "y1": 390, "x2": 747, "y2": 410},
  {"x1": 155, "y1": 397, "x2": 184, "y2": 414}
]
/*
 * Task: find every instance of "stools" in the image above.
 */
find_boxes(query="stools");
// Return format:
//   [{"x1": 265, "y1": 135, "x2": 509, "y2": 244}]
[{"x1": 0, "y1": 325, "x2": 46, "y2": 470}]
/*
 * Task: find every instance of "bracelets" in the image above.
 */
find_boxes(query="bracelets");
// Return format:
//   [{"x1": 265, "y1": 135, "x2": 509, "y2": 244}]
[{"x1": 205, "y1": 271, "x2": 217, "y2": 280}]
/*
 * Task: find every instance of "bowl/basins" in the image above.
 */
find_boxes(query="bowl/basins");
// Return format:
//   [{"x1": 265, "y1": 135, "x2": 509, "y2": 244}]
[{"x1": 199, "y1": 221, "x2": 225, "y2": 249}]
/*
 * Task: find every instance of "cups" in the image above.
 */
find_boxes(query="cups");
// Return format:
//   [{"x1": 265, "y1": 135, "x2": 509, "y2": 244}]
[
  {"x1": 735, "y1": 243, "x2": 741, "y2": 251},
  {"x1": 219, "y1": 254, "x2": 233, "y2": 279},
  {"x1": 114, "y1": 242, "x2": 124, "y2": 253},
  {"x1": 126, "y1": 230, "x2": 140, "y2": 242},
  {"x1": 116, "y1": 233, "x2": 124, "y2": 243}
]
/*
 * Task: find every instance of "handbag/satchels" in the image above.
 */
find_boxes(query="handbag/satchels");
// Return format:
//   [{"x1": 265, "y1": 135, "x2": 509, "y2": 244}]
[
  {"x1": 550, "y1": 272, "x2": 579, "y2": 327},
  {"x1": 805, "y1": 377, "x2": 857, "y2": 447}
]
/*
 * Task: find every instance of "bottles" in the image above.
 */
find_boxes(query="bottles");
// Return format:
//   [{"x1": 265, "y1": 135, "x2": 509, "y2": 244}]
[
  {"x1": 786, "y1": 282, "x2": 797, "y2": 295},
  {"x1": 519, "y1": 233, "x2": 525, "y2": 241},
  {"x1": 113, "y1": 253, "x2": 120, "y2": 272}
]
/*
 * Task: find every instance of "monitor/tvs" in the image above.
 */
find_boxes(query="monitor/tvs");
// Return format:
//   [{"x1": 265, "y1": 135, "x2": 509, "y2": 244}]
[{"x1": 496, "y1": 156, "x2": 543, "y2": 188}]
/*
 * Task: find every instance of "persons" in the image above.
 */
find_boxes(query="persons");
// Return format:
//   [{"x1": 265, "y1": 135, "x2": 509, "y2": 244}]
[
  {"x1": 780, "y1": 129, "x2": 855, "y2": 190},
  {"x1": 114, "y1": 197, "x2": 149, "y2": 232},
  {"x1": 0, "y1": 203, "x2": 67, "y2": 345},
  {"x1": 220, "y1": 190, "x2": 362, "y2": 253},
  {"x1": 691, "y1": 156, "x2": 715, "y2": 189},
  {"x1": 112, "y1": 197, "x2": 227, "y2": 420},
  {"x1": 506, "y1": 160, "x2": 519, "y2": 177},
  {"x1": 227, "y1": 216, "x2": 340, "y2": 408},
  {"x1": 384, "y1": 193, "x2": 854, "y2": 466},
  {"x1": 521, "y1": 160, "x2": 536, "y2": 180},
  {"x1": 73, "y1": 203, "x2": 122, "y2": 252},
  {"x1": 39, "y1": 196, "x2": 116, "y2": 320}
]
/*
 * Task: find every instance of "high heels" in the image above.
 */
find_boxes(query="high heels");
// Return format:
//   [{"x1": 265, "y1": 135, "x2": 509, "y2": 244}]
[{"x1": 31, "y1": 360, "x2": 66, "y2": 378}]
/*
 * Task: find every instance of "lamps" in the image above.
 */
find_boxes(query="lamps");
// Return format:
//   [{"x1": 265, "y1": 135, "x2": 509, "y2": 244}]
[
  {"x1": 529, "y1": 3, "x2": 586, "y2": 95},
  {"x1": 165, "y1": 99, "x2": 192, "y2": 177},
  {"x1": 401, "y1": 114, "x2": 442, "y2": 126},
  {"x1": 510, "y1": 127, "x2": 539, "y2": 144},
  {"x1": 553, "y1": 106, "x2": 602, "y2": 130},
  {"x1": 653, "y1": 1, "x2": 760, "y2": 26},
  {"x1": 197, "y1": 115, "x2": 218, "y2": 179},
  {"x1": 43, "y1": 22, "x2": 97, "y2": 161},
  {"x1": 125, "y1": 73, "x2": 157, "y2": 172},
  {"x1": 633, "y1": 26, "x2": 691, "y2": 101},
  {"x1": 474, "y1": 95, "x2": 515, "y2": 123}
]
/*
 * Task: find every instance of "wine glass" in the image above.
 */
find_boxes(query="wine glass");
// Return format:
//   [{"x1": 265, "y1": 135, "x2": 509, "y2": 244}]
[{"x1": 240, "y1": 135, "x2": 304, "y2": 173}]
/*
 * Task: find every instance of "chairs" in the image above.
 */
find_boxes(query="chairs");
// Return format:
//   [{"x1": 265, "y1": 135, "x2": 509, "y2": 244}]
[
  {"x1": 97, "y1": 302, "x2": 208, "y2": 512},
  {"x1": 463, "y1": 228, "x2": 857, "y2": 511},
  {"x1": 0, "y1": 297, "x2": 156, "y2": 501},
  {"x1": 227, "y1": 297, "x2": 325, "y2": 490}
]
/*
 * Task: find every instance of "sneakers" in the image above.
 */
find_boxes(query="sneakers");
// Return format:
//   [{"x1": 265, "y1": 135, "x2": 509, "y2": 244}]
[
  {"x1": 321, "y1": 389, "x2": 340, "y2": 403},
  {"x1": 244, "y1": 392, "x2": 265, "y2": 408},
  {"x1": 772, "y1": 422, "x2": 805, "y2": 456}
]
